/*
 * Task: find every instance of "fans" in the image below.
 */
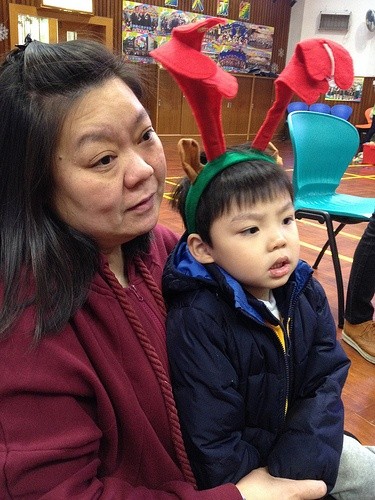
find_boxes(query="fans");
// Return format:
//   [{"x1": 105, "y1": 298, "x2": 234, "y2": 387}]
[{"x1": 365, "y1": 9, "x2": 375, "y2": 32}]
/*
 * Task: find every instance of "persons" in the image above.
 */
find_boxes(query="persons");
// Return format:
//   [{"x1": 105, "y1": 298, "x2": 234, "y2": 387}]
[
  {"x1": 0, "y1": 39, "x2": 361, "y2": 500},
  {"x1": 131, "y1": 5, "x2": 151, "y2": 29},
  {"x1": 341, "y1": 210, "x2": 375, "y2": 365},
  {"x1": 161, "y1": 145, "x2": 375, "y2": 500}
]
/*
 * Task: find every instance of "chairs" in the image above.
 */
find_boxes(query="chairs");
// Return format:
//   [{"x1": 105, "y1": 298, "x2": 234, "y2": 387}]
[{"x1": 284, "y1": 102, "x2": 375, "y2": 329}]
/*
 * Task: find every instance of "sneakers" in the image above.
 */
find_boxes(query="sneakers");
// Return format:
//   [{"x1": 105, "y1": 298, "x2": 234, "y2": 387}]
[{"x1": 341, "y1": 317, "x2": 375, "y2": 363}]
